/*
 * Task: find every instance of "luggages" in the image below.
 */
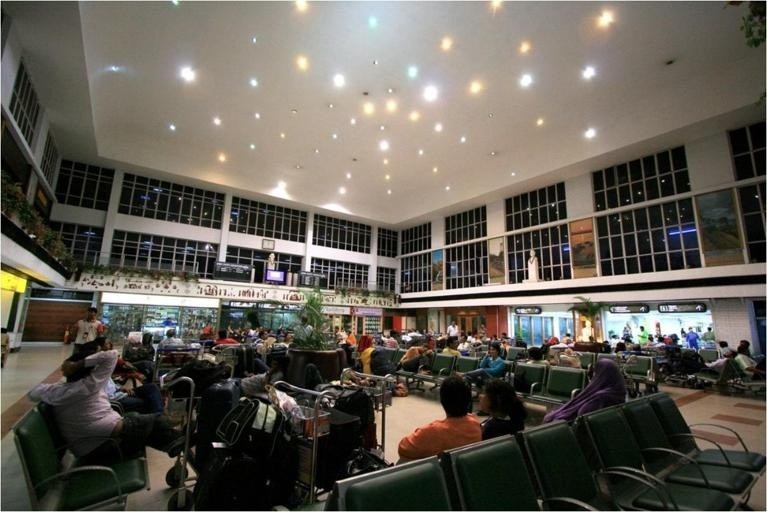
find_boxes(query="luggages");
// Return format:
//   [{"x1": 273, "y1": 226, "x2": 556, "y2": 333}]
[
  {"x1": 194, "y1": 440, "x2": 244, "y2": 510},
  {"x1": 237, "y1": 345, "x2": 255, "y2": 377},
  {"x1": 200, "y1": 377, "x2": 241, "y2": 443}
]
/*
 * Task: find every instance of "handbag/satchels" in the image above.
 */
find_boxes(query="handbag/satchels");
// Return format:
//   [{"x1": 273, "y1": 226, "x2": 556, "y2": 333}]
[{"x1": 314, "y1": 387, "x2": 369, "y2": 439}]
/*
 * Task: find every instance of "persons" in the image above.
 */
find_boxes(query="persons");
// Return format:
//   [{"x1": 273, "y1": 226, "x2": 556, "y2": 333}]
[
  {"x1": 455, "y1": 342, "x2": 506, "y2": 418},
  {"x1": 470, "y1": 382, "x2": 534, "y2": 441},
  {"x1": 395, "y1": 376, "x2": 484, "y2": 465},
  {"x1": 64, "y1": 304, "x2": 766, "y2": 396},
  {"x1": 527, "y1": 248, "x2": 540, "y2": 279},
  {"x1": 541, "y1": 357, "x2": 627, "y2": 426},
  {"x1": 77, "y1": 340, "x2": 165, "y2": 416},
  {"x1": 27, "y1": 348, "x2": 196, "y2": 471}
]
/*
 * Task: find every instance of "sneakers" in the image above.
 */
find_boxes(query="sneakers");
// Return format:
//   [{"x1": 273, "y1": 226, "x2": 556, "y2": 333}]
[{"x1": 166, "y1": 431, "x2": 197, "y2": 458}]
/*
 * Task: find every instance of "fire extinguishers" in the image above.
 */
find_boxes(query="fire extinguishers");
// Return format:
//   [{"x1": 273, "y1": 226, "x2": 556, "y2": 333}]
[{"x1": 64, "y1": 327, "x2": 72, "y2": 344}]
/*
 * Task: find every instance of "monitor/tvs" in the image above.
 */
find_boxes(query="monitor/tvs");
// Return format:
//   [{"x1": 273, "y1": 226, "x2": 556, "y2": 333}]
[{"x1": 266, "y1": 269, "x2": 285, "y2": 284}]
